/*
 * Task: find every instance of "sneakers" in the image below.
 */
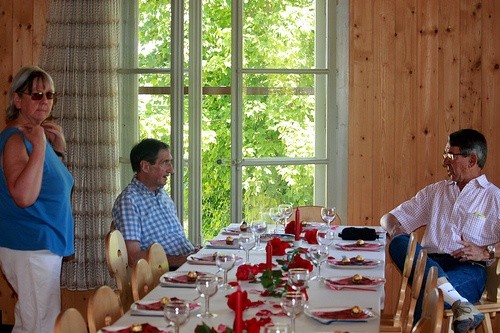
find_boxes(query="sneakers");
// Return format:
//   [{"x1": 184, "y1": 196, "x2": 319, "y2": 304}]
[{"x1": 451, "y1": 300, "x2": 485, "y2": 333}]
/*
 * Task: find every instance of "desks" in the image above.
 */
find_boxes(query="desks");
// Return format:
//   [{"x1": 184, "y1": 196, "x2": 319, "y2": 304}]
[{"x1": 100, "y1": 224, "x2": 386, "y2": 333}]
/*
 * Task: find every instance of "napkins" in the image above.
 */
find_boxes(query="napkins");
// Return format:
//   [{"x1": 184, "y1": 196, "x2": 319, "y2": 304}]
[
  {"x1": 327, "y1": 256, "x2": 378, "y2": 267},
  {"x1": 101, "y1": 321, "x2": 171, "y2": 333},
  {"x1": 326, "y1": 275, "x2": 383, "y2": 285},
  {"x1": 311, "y1": 306, "x2": 370, "y2": 323},
  {"x1": 133, "y1": 300, "x2": 202, "y2": 310},
  {"x1": 164, "y1": 272, "x2": 217, "y2": 284},
  {"x1": 188, "y1": 225, "x2": 255, "y2": 265},
  {"x1": 334, "y1": 242, "x2": 383, "y2": 250}
]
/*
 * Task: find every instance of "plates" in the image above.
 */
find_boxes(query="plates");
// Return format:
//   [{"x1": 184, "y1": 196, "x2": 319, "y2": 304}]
[
  {"x1": 305, "y1": 305, "x2": 376, "y2": 325},
  {"x1": 336, "y1": 243, "x2": 383, "y2": 250},
  {"x1": 328, "y1": 259, "x2": 379, "y2": 268},
  {"x1": 220, "y1": 227, "x2": 239, "y2": 234},
  {"x1": 98, "y1": 326, "x2": 163, "y2": 333},
  {"x1": 327, "y1": 275, "x2": 386, "y2": 288},
  {"x1": 203, "y1": 238, "x2": 240, "y2": 249},
  {"x1": 130, "y1": 300, "x2": 203, "y2": 316},
  {"x1": 186, "y1": 254, "x2": 244, "y2": 264},
  {"x1": 159, "y1": 270, "x2": 223, "y2": 287}
]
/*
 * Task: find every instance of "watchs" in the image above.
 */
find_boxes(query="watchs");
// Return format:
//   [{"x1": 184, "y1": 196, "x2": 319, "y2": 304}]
[
  {"x1": 486, "y1": 245, "x2": 496, "y2": 259},
  {"x1": 54, "y1": 149, "x2": 65, "y2": 157}
]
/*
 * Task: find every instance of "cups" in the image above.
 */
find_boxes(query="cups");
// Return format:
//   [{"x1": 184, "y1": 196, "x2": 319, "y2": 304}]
[{"x1": 265, "y1": 321, "x2": 290, "y2": 333}]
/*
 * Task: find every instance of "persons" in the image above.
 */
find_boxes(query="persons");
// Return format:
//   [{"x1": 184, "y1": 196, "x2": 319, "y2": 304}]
[
  {"x1": 0, "y1": 64, "x2": 74, "y2": 333},
  {"x1": 111, "y1": 138, "x2": 202, "y2": 271},
  {"x1": 380, "y1": 127, "x2": 500, "y2": 333}
]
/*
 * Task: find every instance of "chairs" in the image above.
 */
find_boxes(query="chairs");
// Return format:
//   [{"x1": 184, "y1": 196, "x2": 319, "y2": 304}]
[
  {"x1": 286, "y1": 204, "x2": 339, "y2": 226},
  {"x1": 381, "y1": 231, "x2": 500, "y2": 333},
  {"x1": 53, "y1": 229, "x2": 170, "y2": 333}
]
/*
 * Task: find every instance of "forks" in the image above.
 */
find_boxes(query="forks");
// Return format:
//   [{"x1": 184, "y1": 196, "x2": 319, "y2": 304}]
[{"x1": 311, "y1": 317, "x2": 368, "y2": 325}]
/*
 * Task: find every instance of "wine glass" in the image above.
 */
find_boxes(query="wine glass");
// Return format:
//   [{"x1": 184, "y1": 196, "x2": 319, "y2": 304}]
[
  {"x1": 280, "y1": 207, "x2": 336, "y2": 333},
  {"x1": 164, "y1": 204, "x2": 293, "y2": 333}
]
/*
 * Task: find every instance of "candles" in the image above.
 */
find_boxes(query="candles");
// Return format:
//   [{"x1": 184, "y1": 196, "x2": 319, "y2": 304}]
[
  {"x1": 266, "y1": 240, "x2": 273, "y2": 270},
  {"x1": 295, "y1": 208, "x2": 299, "y2": 240},
  {"x1": 234, "y1": 286, "x2": 243, "y2": 333}
]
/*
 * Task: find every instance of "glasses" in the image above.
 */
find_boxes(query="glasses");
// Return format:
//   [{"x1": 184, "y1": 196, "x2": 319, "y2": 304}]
[
  {"x1": 442, "y1": 152, "x2": 470, "y2": 161},
  {"x1": 22, "y1": 92, "x2": 56, "y2": 100}
]
